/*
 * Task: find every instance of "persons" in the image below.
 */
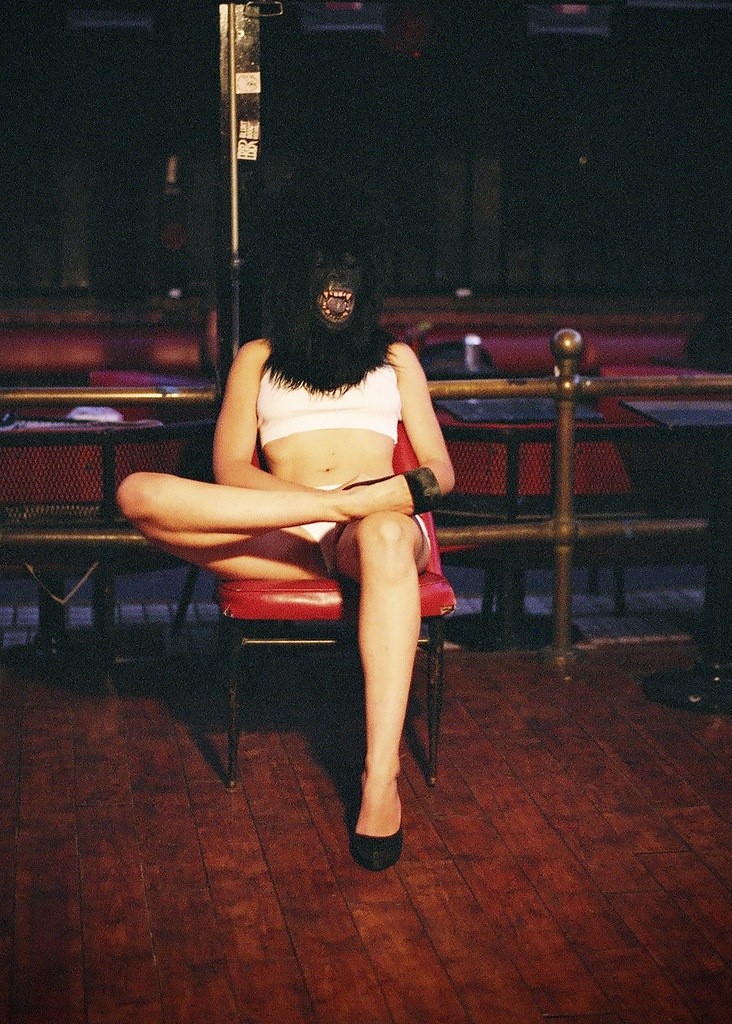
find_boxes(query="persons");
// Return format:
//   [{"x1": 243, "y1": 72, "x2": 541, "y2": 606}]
[{"x1": 114, "y1": 238, "x2": 456, "y2": 871}]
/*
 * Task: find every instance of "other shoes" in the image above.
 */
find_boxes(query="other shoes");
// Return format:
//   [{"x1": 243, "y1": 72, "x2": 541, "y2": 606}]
[
  {"x1": 347, "y1": 767, "x2": 405, "y2": 872},
  {"x1": 343, "y1": 465, "x2": 444, "y2": 516}
]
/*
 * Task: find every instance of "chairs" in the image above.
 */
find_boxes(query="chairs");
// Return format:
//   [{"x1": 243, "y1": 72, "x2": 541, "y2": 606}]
[{"x1": 217, "y1": 423, "x2": 459, "y2": 790}]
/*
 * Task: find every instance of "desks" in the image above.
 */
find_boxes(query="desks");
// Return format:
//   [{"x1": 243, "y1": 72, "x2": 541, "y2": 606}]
[
  {"x1": 619, "y1": 401, "x2": 732, "y2": 711},
  {"x1": 438, "y1": 401, "x2": 606, "y2": 653},
  {"x1": 0, "y1": 419, "x2": 213, "y2": 691}
]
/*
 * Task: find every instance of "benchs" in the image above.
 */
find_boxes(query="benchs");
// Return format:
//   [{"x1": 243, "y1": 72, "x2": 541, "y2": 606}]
[{"x1": 0, "y1": 328, "x2": 732, "y2": 652}]
